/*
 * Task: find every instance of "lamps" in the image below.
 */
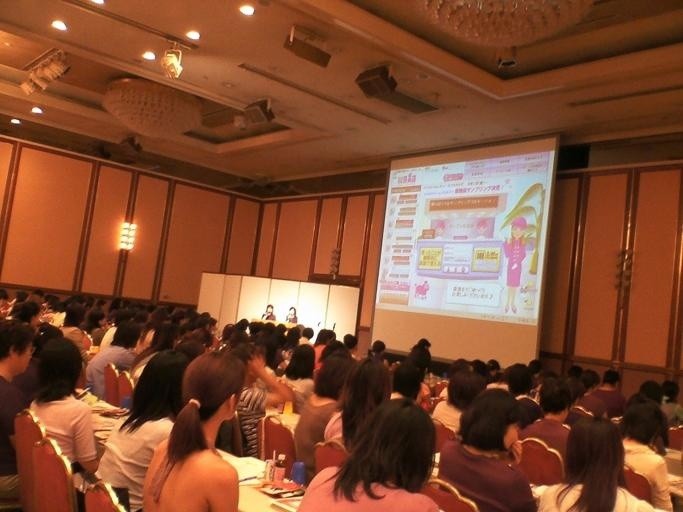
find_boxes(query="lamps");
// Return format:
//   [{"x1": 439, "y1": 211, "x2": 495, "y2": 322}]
[
  {"x1": 613, "y1": 248, "x2": 633, "y2": 288},
  {"x1": 160, "y1": 41, "x2": 183, "y2": 78},
  {"x1": 420, "y1": 0, "x2": 594, "y2": 48},
  {"x1": 243, "y1": 96, "x2": 276, "y2": 124},
  {"x1": 120, "y1": 223, "x2": 137, "y2": 250},
  {"x1": 103, "y1": 78, "x2": 204, "y2": 140},
  {"x1": 283, "y1": 23, "x2": 330, "y2": 67},
  {"x1": 330, "y1": 247, "x2": 341, "y2": 274},
  {"x1": 20, "y1": 48, "x2": 73, "y2": 96}
]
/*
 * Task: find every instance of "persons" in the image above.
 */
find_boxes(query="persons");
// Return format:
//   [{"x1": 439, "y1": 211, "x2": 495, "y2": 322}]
[{"x1": 0, "y1": 287, "x2": 683, "y2": 512}]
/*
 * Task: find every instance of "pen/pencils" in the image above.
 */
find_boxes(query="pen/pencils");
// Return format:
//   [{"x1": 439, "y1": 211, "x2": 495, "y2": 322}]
[{"x1": 238, "y1": 476, "x2": 257, "y2": 482}]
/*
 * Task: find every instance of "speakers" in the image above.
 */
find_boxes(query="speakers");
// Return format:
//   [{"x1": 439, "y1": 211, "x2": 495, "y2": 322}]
[{"x1": 244, "y1": 101, "x2": 275, "y2": 126}]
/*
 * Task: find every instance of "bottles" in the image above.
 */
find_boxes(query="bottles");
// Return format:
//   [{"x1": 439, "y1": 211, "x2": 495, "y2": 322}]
[{"x1": 264, "y1": 453, "x2": 306, "y2": 486}]
